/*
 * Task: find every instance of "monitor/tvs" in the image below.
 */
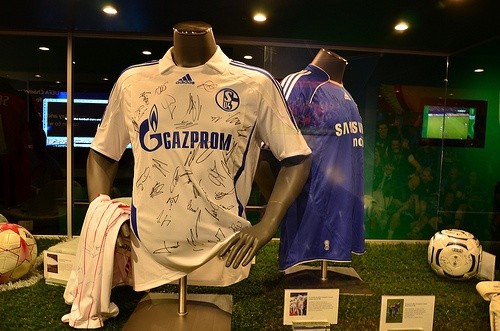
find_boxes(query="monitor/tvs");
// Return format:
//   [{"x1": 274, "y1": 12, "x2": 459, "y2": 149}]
[
  {"x1": 42, "y1": 95, "x2": 132, "y2": 150},
  {"x1": 417, "y1": 98, "x2": 488, "y2": 148}
]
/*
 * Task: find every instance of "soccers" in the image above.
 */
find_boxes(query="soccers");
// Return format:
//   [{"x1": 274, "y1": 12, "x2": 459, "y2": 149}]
[
  {"x1": 428, "y1": 229, "x2": 483, "y2": 280},
  {"x1": 0, "y1": 224, "x2": 39, "y2": 284}
]
